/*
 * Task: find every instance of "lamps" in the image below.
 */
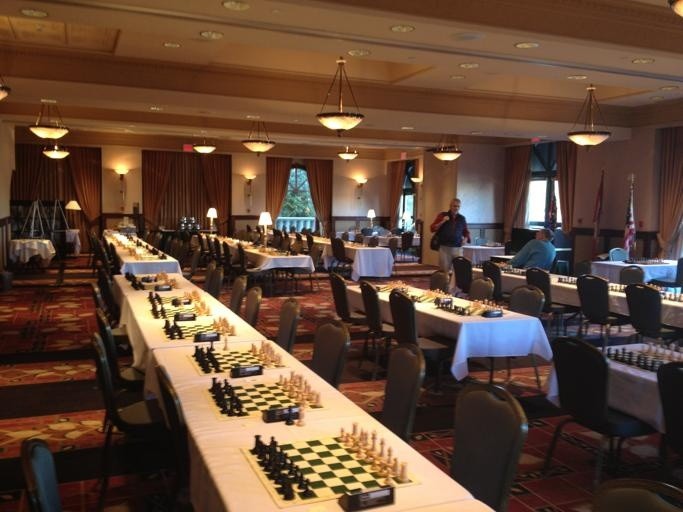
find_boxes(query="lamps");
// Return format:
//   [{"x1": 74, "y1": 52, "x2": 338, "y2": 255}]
[
  {"x1": 433, "y1": 136, "x2": 463, "y2": 161},
  {"x1": 367, "y1": 209, "x2": 375, "y2": 228},
  {"x1": 337, "y1": 146, "x2": 358, "y2": 161},
  {"x1": 65, "y1": 200, "x2": 82, "y2": 229},
  {"x1": 0, "y1": 73, "x2": 11, "y2": 100},
  {"x1": 192, "y1": 138, "x2": 216, "y2": 154},
  {"x1": 115, "y1": 170, "x2": 129, "y2": 212},
  {"x1": 568, "y1": 84, "x2": 612, "y2": 151},
  {"x1": 28, "y1": 100, "x2": 70, "y2": 139},
  {"x1": 316, "y1": 55, "x2": 364, "y2": 136},
  {"x1": 243, "y1": 175, "x2": 256, "y2": 212},
  {"x1": 410, "y1": 177, "x2": 422, "y2": 233},
  {"x1": 258, "y1": 212, "x2": 273, "y2": 236},
  {"x1": 669, "y1": 0, "x2": 682, "y2": 16},
  {"x1": 42, "y1": 139, "x2": 69, "y2": 160},
  {"x1": 241, "y1": 121, "x2": 276, "y2": 156},
  {"x1": 355, "y1": 179, "x2": 368, "y2": 199},
  {"x1": 206, "y1": 208, "x2": 218, "y2": 229}
]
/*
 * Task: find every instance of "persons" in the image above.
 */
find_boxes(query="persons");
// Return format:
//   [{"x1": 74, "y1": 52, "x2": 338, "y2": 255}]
[
  {"x1": 430, "y1": 198, "x2": 470, "y2": 283},
  {"x1": 508, "y1": 228, "x2": 557, "y2": 272}
]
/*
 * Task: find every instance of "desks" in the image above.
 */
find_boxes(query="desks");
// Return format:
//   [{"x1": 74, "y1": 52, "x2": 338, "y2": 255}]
[{"x1": 7, "y1": 239, "x2": 56, "y2": 273}]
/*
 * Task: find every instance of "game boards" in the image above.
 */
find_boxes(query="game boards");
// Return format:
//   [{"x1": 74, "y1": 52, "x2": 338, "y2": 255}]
[
  {"x1": 130, "y1": 281, "x2": 183, "y2": 291},
  {"x1": 496, "y1": 261, "x2": 547, "y2": 277},
  {"x1": 150, "y1": 306, "x2": 211, "y2": 319},
  {"x1": 126, "y1": 271, "x2": 168, "y2": 282},
  {"x1": 624, "y1": 257, "x2": 668, "y2": 265},
  {"x1": 160, "y1": 319, "x2": 236, "y2": 342},
  {"x1": 603, "y1": 343, "x2": 682, "y2": 371},
  {"x1": 375, "y1": 281, "x2": 505, "y2": 316},
  {"x1": 187, "y1": 342, "x2": 289, "y2": 378},
  {"x1": 202, "y1": 233, "x2": 298, "y2": 257},
  {"x1": 146, "y1": 290, "x2": 201, "y2": 306},
  {"x1": 107, "y1": 231, "x2": 167, "y2": 261},
  {"x1": 206, "y1": 371, "x2": 324, "y2": 421},
  {"x1": 558, "y1": 277, "x2": 683, "y2": 302},
  {"x1": 240, "y1": 422, "x2": 422, "y2": 510}
]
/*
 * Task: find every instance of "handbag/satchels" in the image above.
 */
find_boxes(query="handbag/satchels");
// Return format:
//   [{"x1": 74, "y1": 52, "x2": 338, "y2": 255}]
[{"x1": 430, "y1": 222, "x2": 445, "y2": 251}]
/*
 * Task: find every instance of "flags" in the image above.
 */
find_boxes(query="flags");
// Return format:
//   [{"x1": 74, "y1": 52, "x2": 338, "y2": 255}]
[
  {"x1": 548, "y1": 189, "x2": 557, "y2": 231},
  {"x1": 624, "y1": 185, "x2": 636, "y2": 253},
  {"x1": 590, "y1": 177, "x2": 603, "y2": 260}
]
